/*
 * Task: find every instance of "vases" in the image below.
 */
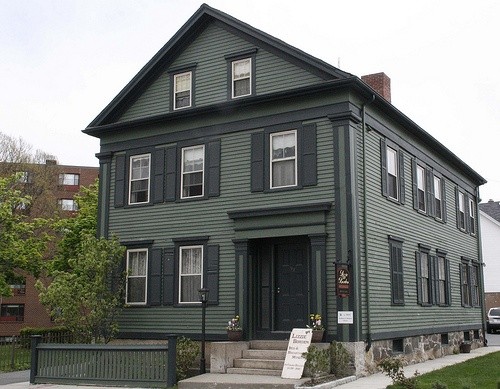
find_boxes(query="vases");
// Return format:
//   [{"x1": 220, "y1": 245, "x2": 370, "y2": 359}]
[
  {"x1": 311, "y1": 328, "x2": 325, "y2": 342},
  {"x1": 227, "y1": 329, "x2": 242, "y2": 341}
]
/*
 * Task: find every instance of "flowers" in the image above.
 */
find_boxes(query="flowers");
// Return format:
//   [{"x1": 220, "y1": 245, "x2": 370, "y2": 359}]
[
  {"x1": 309, "y1": 313, "x2": 323, "y2": 330},
  {"x1": 226, "y1": 315, "x2": 242, "y2": 329}
]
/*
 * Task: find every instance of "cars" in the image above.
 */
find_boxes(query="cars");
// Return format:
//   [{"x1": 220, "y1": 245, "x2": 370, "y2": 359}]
[{"x1": 486, "y1": 308, "x2": 500, "y2": 333}]
